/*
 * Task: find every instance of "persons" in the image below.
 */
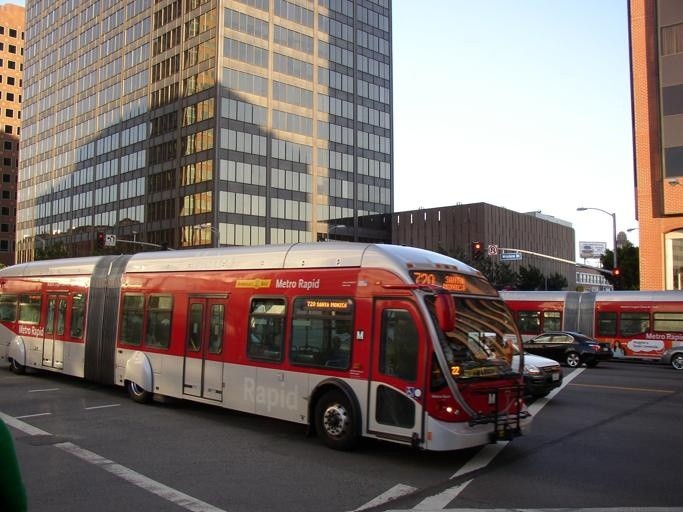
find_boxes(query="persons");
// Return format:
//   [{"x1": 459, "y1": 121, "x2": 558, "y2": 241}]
[
  {"x1": 444, "y1": 328, "x2": 478, "y2": 367},
  {"x1": 323, "y1": 337, "x2": 346, "y2": 367},
  {"x1": 610, "y1": 340, "x2": 627, "y2": 356}
]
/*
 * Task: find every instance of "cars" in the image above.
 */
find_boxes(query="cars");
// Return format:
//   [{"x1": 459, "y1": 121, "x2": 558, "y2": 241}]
[
  {"x1": 521, "y1": 331, "x2": 613, "y2": 367},
  {"x1": 473, "y1": 334, "x2": 562, "y2": 402}
]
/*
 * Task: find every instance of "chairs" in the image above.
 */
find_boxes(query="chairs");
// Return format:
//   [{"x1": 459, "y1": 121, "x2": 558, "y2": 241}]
[{"x1": 263, "y1": 345, "x2": 347, "y2": 367}]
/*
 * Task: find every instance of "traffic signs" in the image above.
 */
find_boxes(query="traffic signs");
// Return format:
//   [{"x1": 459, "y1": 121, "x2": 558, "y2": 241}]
[{"x1": 500, "y1": 253, "x2": 522, "y2": 260}]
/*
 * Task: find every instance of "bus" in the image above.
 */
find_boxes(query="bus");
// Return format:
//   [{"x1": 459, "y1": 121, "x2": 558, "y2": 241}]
[
  {"x1": 499, "y1": 292, "x2": 683, "y2": 369},
  {"x1": 1, "y1": 241, "x2": 531, "y2": 452}
]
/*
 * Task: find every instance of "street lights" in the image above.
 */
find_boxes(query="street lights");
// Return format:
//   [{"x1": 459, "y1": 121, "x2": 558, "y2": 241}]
[{"x1": 576, "y1": 208, "x2": 621, "y2": 290}]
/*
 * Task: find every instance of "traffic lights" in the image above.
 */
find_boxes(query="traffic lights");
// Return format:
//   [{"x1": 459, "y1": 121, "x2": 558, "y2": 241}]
[
  {"x1": 613, "y1": 268, "x2": 620, "y2": 276},
  {"x1": 97, "y1": 231, "x2": 104, "y2": 248},
  {"x1": 472, "y1": 242, "x2": 483, "y2": 261}
]
